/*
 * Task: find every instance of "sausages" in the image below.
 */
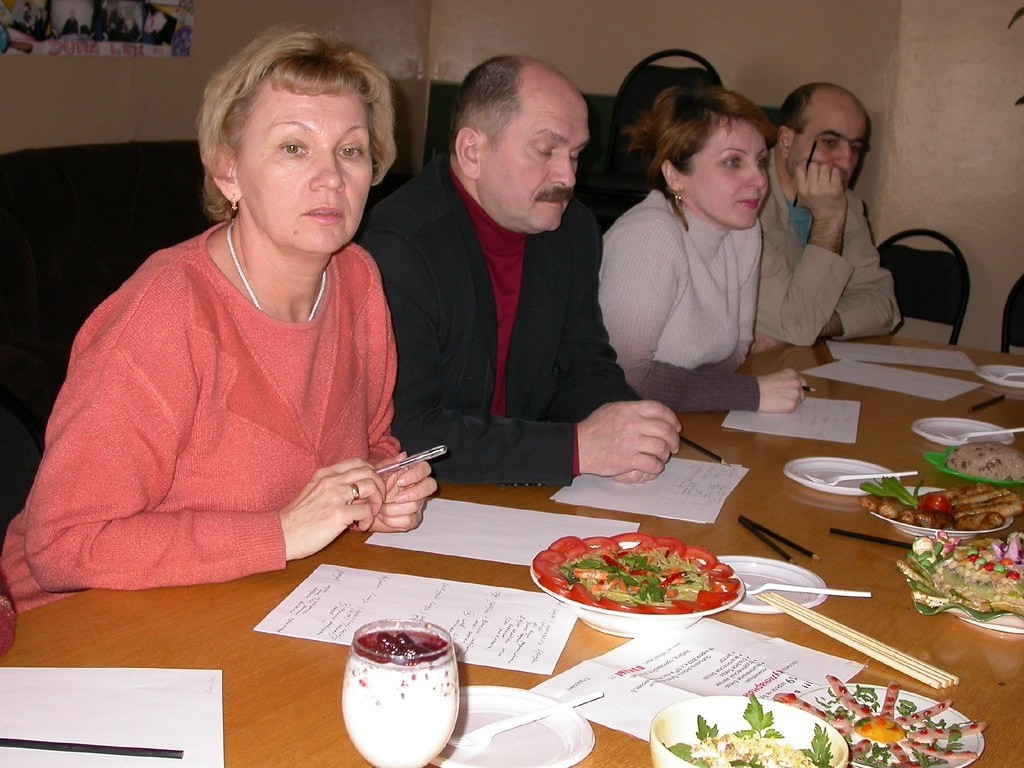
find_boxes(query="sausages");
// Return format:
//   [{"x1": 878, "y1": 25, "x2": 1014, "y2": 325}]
[
  {"x1": 916, "y1": 483, "x2": 1024, "y2": 531},
  {"x1": 859, "y1": 494, "x2": 954, "y2": 529}
]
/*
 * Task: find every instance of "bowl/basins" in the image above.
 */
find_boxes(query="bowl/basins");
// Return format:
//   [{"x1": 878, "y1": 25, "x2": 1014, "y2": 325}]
[{"x1": 649, "y1": 696, "x2": 849, "y2": 768}]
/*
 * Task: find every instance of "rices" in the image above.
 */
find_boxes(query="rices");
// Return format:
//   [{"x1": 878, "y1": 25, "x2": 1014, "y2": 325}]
[{"x1": 945, "y1": 441, "x2": 1024, "y2": 481}]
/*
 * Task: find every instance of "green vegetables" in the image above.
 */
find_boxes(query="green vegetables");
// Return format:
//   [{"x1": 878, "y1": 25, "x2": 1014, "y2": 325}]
[
  {"x1": 660, "y1": 689, "x2": 834, "y2": 768},
  {"x1": 859, "y1": 474, "x2": 925, "y2": 508},
  {"x1": 813, "y1": 686, "x2": 978, "y2": 768}
]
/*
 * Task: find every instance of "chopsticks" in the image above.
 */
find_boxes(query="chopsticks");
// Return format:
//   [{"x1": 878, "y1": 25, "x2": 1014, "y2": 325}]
[{"x1": 754, "y1": 592, "x2": 960, "y2": 689}]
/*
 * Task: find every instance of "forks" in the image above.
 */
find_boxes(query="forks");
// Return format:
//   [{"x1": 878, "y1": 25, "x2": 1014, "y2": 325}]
[
  {"x1": 447, "y1": 691, "x2": 607, "y2": 750},
  {"x1": 803, "y1": 469, "x2": 918, "y2": 486},
  {"x1": 983, "y1": 372, "x2": 1024, "y2": 379}
]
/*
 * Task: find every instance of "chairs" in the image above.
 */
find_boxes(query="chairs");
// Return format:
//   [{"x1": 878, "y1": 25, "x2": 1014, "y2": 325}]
[
  {"x1": 876, "y1": 228, "x2": 970, "y2": 346},
  {"x1": 0, "y1": 403, "x2": 45, "y2": 542},
  {"x1": 1000, "y1": 274, "x2": 1024, "y2": 352},
  {"x1": 582, "y1": 50, "x2": 722, "y2": 228}
]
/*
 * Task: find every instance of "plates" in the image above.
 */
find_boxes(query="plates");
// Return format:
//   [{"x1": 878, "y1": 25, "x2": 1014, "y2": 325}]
[
  {"x1": 530, "y1": 546, "x2": 745, "y2": 638},
  {"x1": 782, "y1": 457, "x2": 901, "y2": 496},
  {"x1": 792, "y1": 683, "x2": 986, "y2": 768},
  {"x1": 922, "y1": 445, "x2": 1024, "y2": 488},
  {"x1": 911, "y1": 416, "x2": 1015, "y2": 450},
  {"x1": 975, "y1": 364, "x2": 1024, "y2": 389},
  {"x1": 424, "y1": 685, "x2": 596, "y2": 767},
  {"x1": 862, "y1": 485, "x2": 1014, "y2": 540},
  {"x1": 708, "y1": 554, "x2": 829, "y2": 615},
  {"x1": 894, "y1": 532, "x2": 1024, "y2": 634}
]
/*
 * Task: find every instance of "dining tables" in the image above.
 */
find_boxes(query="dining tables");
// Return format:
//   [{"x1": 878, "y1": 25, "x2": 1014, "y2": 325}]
[{"x1": 0, "y1": 332, "x2": 1024, "y2": 768}]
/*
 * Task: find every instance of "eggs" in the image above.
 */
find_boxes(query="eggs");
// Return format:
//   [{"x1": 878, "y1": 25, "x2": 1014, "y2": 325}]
[{"x1": 854, "y1": 717, "x2": 906, "y2": 744}]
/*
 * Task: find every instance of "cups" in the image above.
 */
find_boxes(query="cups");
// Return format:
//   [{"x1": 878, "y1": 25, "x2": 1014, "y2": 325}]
[{"x1": 341, "y1": 619, "x2": 459, "y2": 768}]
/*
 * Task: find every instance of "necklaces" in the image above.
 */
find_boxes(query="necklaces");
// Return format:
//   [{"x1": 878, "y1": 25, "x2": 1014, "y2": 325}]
[{"x1": 227, "y1": 223, "x2": 326, "y2": 321}]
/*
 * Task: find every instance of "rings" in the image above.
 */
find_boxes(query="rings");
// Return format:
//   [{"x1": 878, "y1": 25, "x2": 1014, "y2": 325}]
[
  {"x1": 419, "y1": 498, "x2": 425, "y2": 510},
  {"x1": 351, "y1": 484, "x2": 359, "y2": 501}
]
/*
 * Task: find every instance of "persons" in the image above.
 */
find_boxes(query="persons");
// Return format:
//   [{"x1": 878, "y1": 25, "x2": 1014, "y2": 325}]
[
  {"x1": 747, "y1": 82, "x2": 902, "y2": 353},
  {"x1": 11, "y1": 2, "x2": 43, "y2": 39},
  {"x1": 81, "y1": 10, "x2": 166, "y2": 44},
  {"x1": 598, "y1": 85, "x2": 808, "y2": 414},
  {"x1": 350, "y1": 54, "x2": 681, "y2": 485},
  {"x1": 0, "y1": 24, "x2": 436, "y2": 614},
  {"x1": 60, "y1": 9, "x2": 79, "y2": 36}
]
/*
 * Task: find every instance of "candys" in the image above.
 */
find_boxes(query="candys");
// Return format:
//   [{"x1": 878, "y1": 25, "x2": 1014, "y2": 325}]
[{"x1": 966, "y1": 547, "x2": 1020, "y2": 580}]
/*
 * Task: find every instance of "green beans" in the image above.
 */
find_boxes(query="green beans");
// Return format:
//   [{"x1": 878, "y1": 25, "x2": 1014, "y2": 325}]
[{"x1": 901, "y1": 534, "x2": 962, "y2": 597}]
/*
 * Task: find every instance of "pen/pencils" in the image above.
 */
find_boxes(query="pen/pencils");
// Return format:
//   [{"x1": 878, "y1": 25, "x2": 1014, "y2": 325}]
[
  {"x1": 375, "y1": 445, "x2": 448, "y2": 474},
  {"x1": 680, "y1": 435, "x2": 731, "y2": 467},
  {"x1": 802, "y1": 386, "x2": 817, "y2": 392},
  {"x1": 792, "y1": 139, "x2": 819, "y2": 207},
  {"x1": 969, "y1": 394, "x2": 1005, "y2": 412},
  {"x1": 738, "y1": 517, "x2": 796, "y2": 564},
  {"x1": 741, "y1": 515, "x2": 820, "y2": 560},
  {"x1": 0, "y1": 737, "x2": 186, "y2": 760},
  {"x1": 830, "y1": 527, "x2": 913, "y2": 548}
]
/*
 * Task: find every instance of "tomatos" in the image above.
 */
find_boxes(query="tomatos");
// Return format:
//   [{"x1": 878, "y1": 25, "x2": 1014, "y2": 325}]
[
  {"x1": 918, "y1": 493, "x2": 952, "y2": 511},
  {"x1": 532, "y1": 532, "x2": 740, "y2": 613}
]
